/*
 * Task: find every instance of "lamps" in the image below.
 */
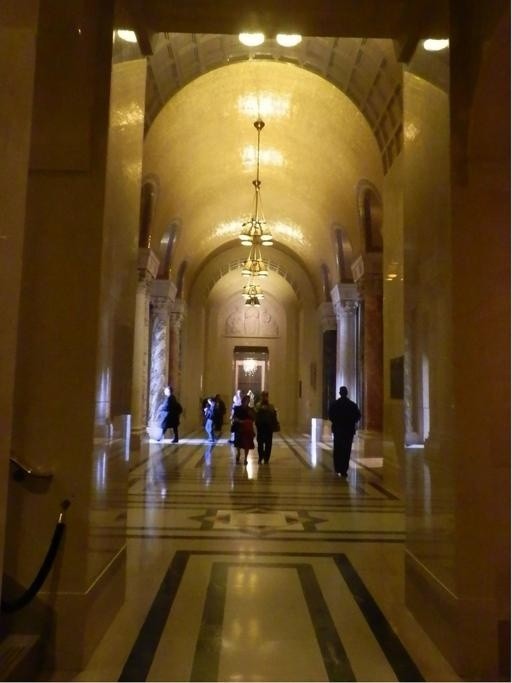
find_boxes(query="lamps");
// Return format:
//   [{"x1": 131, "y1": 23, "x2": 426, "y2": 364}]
[{"x1": 239, "y1": 95, "x2": 274, "y2": 309}]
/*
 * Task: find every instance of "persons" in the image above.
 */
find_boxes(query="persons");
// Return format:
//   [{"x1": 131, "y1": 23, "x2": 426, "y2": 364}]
[
  {"x1": 214, "y1": 393, "x2": 226, "y2": 431},
  {"x1": 326, "y1": 385, "x2": 360, "y2": 477},
  {"x1": 203, "y1": 397, "x2": 218, "y2": 442},
  {"x1": 230, "y1": 396, "x2": 256, "y2": 465},
  {"x1": 256, "y1": 391, "x2": 280, "y2": 465},
  {"x1": 156, "y1": 386, "x2": 183, "y2": 443}
]
